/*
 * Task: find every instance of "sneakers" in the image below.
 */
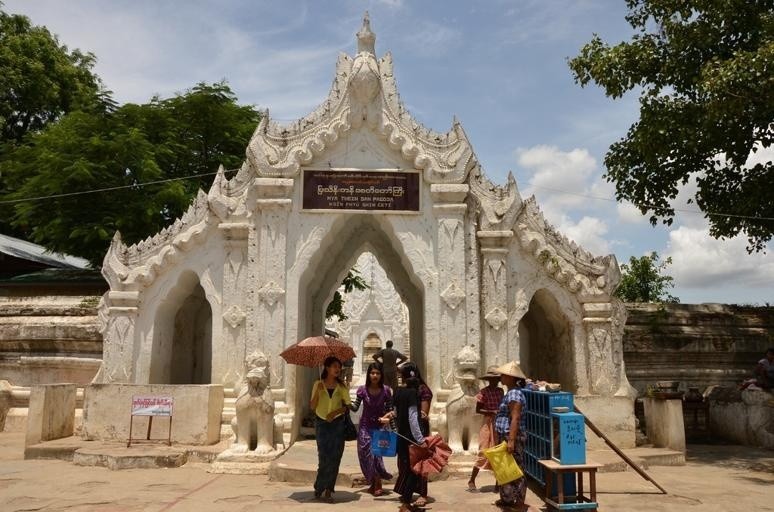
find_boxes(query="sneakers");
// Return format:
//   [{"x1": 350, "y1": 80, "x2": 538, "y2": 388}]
[
  {"x1": 312, "y1": 487, "x2": 334, "y2": 503},
  {"x1": 492, "y1": 498, "x2": 525, "y2": 511},
  {"x1": 365, "y1": 478, "x2": 429, "y2": 507}
]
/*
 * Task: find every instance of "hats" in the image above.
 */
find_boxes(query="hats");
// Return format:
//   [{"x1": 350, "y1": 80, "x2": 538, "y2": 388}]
[
  {"x1": 479, "y1": 364, "x2": 503, "y2": 379},
  {"x1": 493, "y1": 360, "x2": 527, "y2": 380}
]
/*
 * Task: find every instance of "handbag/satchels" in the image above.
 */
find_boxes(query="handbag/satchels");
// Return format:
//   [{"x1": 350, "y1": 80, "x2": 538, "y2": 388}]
[
  {"x1": 479, "y1": 439, "x2": 524, "y2": 487},
  {"x1": 342, "y1": 405, "x2": 357, "y2": 442},
  {"x1": 370, "y1": 422, "x2": 399, "y2": 458}
]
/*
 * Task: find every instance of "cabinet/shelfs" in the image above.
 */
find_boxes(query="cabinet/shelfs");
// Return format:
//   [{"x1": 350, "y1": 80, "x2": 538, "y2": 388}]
[{"x1": 519, "y1": 387, "x2": 584, "y2": 502}]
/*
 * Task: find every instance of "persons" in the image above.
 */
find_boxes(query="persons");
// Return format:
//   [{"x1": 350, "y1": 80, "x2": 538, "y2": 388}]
[
  {"x1": 372, "y1": 341, "x2": 409, "y2": 392},
  {"x1": 391, "y1": 365, "x2": 428, "y2": 512},
  {"x1": 756, "y1": 348, "x2": 773, "y2": 393},
  {"x1": 341, "y1": 359, "x2": 355, "y2": 389},
  {"x1": 467, "y1": 365, "x2": 505, "y2": 492},
  {"x1": 346, "y1": 362, "x2": 393, "y2": 496},
  {"x1": 385, "y1": 361, "x2": 434, "y2": 506},
  {"x1": 494, "y1": 359, "x2": 530, "y2": 512},
  {"x1": 309, "y1": 357, "x2": 351, "y2": 503}
]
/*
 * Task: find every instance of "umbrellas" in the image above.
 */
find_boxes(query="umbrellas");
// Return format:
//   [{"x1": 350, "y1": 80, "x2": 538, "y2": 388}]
[
  {"x1": 382, "y1": 424, "x2": 452, "y2": 477},
  {"x1": 277, "y1": 335, "x2": 357, "y2": 385}
]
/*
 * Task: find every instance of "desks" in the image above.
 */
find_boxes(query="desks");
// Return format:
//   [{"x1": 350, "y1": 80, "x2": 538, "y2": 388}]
[{"x1": 536, "y1": 459, "x2": 604, "y2": 512}]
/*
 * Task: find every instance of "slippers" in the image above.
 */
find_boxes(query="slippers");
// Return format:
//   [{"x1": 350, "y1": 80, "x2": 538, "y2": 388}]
[{"x1": 465, "y1": 488, "x2": 483, "y2": 494}]
